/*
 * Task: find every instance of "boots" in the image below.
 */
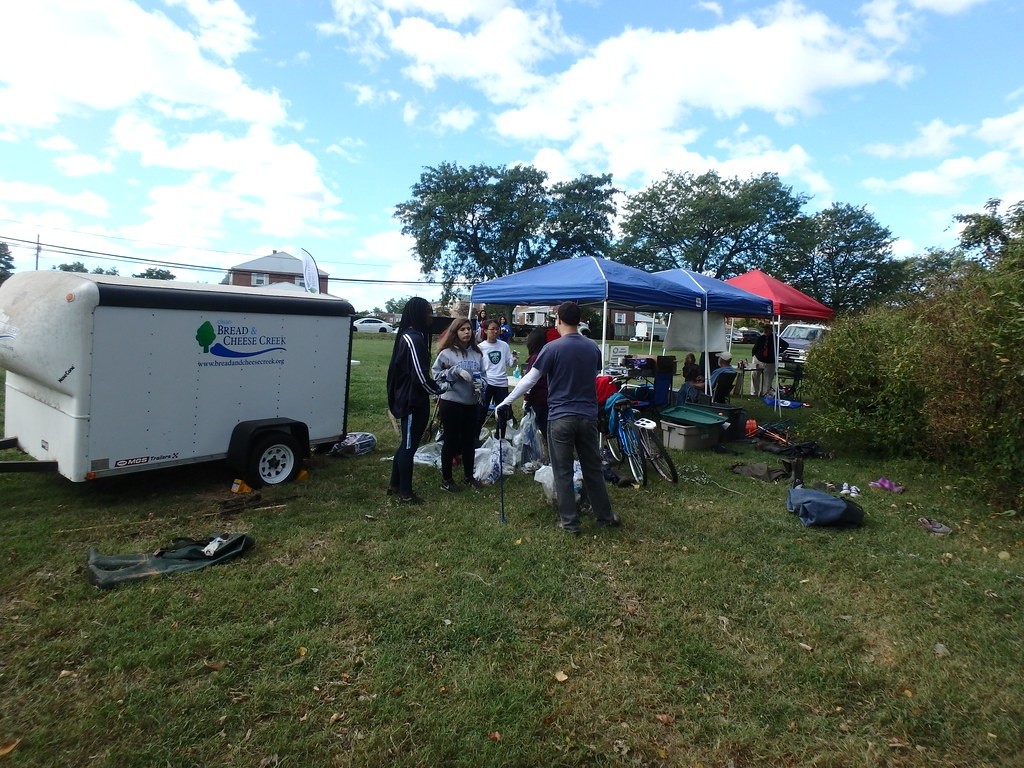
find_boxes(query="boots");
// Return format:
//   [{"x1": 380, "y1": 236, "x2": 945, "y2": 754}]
[
  {"x1": 780, "y1": 459, "x2": 791, "y2": 481},
  {"x1": 613, "y1": 470, "x2": 633, "y2": 488},
  {"x1": 87, "y1": 547, "x2": 154, "y2": 567},
  {"x1": 785, "y1": 459, "x2": 804, "y2": 484},
  {"x1": 88, "y1": 564, "x2": 159, "y2": 587}
]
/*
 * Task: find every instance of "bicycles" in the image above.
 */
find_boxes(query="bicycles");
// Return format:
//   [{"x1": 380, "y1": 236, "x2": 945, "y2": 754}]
[{"x1": 601, "y1": 375, "x2": 680, "y2": 487}]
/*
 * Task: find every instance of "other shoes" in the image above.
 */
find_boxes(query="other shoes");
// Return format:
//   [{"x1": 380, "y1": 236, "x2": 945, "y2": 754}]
[
  {"x1": 610, "y1": 514, "x2": 622, "y2": 525},
  {"x1": 556, "y1": 521, "x2": 578, "y2": 537},
  {"x1": 748, "y1": 395, "x2": 757, "y2": 400},
  {"x1": 869, "y1": 476, "x2": 905, "y2": 494}
]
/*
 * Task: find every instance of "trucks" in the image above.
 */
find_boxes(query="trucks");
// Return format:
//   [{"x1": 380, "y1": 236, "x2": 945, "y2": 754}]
[{"x1": 511, "y1": 313, "x2": 592, "y2": 339}]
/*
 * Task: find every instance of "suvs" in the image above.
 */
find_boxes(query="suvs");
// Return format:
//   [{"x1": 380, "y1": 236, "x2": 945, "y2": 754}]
[{"x1": 777, "y1": 322, "x2": 830, "y2": 373}]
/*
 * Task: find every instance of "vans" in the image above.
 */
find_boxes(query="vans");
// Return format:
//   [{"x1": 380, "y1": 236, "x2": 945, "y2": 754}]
[{"x1": 635, "y1": 322, "x2": 668, "y2": 341}]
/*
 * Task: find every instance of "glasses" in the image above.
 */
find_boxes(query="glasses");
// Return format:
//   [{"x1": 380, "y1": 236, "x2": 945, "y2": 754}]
[
  {"x1": 763, "y1": 326, "x2": 769, "y2": 328},
  {"x1": 488, "y1": 328, "x2": 499, "y2": 331}
]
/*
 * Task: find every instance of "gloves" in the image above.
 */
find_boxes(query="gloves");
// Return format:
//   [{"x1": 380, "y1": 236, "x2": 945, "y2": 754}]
[
  {"x1": 458, "y1": 369, "x2": 472, "y2": 383},
  {"x1": 522, "y1": 400, "x2": 529, "y2": 412},
  {"x1": 495, "y1": 401, "x2": 509, "y2": 421},
  {"x1": 511, "y1": 350, "x2": 520, "y2": 362},
  {"x1": 473, "y1": 378, "x2": 484, "y2": 393}
]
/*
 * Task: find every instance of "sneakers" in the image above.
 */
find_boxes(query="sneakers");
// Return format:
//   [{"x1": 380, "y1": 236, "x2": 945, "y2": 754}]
[
  {"x1": 440, "y1": 480, "x2": 459, "y2": 495},
  {"x1": 463, "y1": 476, "x2": 482, "y2": 489},
  {"x1": 387, "y1": 489, "x2": 400, "y2": 495},
  {"x1": 919, "y1": 517, "x2": 952, "y2": 535},
  {"x1": 400, "y1": 494, "x2": 424, "y2": 505},
  {"x1": 839, "y1": 482, "x2": 850, "y2": 495},
  {"x1": 850, "y1": 485, "x2": 863, "y2": 497}
]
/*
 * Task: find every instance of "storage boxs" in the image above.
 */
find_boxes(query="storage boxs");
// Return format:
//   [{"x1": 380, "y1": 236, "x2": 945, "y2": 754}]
[{"x1": 660, "y1": 419, "x2": 721, "y2": 454}]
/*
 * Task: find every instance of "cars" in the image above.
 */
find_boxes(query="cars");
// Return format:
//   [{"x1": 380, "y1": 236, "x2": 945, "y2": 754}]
[
  {"x1": 725, "y1": 325, "x2": 743, "y2": 344},
  {"x1": 739, "y1": 328, "x2": 762, "y2": 344},
  {"x1": 353, "y1": 317, "x2": 394, "y2": 333}
]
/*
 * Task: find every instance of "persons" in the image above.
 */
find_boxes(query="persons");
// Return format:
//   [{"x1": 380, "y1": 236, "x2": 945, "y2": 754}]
[
  {"x1": 476, "y1": 319, "x2": 520, "y2": 446},
  {"x1": 546, "y1": 317, "x2": 561, "y2": 342},
  {"x1": 524, "y1": 328, "x2": 551, "y2": 442},
  {"x1": 674, "y1": 352, "x2": 737, "y2": 406},
  {"x1": 387, "y1": 297, "x2": 453, "y2": 506},
  {"x1": 748, "y1": 324, "x2": 789, "y2": 397},
  {"x1": 494, "y1": 301, "x2": 620, "y2": 535},
  {"x1": 431, "y1": 317, "x2": 487, "y2": 495},
  {"x1": 474, "y1": 310, "x2": 511, "y2": 344}
]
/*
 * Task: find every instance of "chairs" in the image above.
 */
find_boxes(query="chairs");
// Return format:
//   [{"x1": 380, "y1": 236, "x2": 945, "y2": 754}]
[
  {"x1": 777, "y1": 363, "x2": 804, "y2": 402},
  {"x1": 686, "y1": 369, "x2": 739, "y2": 404},
  {"x1": 683, "y1": 401, "x2": 743, "y2": 433},
  {"x1": 643, "y1": 370, "x2": 674, "y2": 417}
]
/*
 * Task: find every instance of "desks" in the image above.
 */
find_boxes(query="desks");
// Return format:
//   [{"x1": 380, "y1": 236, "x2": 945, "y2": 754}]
[
  {"x1": 731, "y1": 365, "x2": 765, "y2": 398},
  {"x1": 490, "y1": 375, "x2": 522, "y2": 405},
  {"x1": 618, "y1": 373, "x2": 681, "y2": 405},
  {"x1": 632, "y1": 400, "x2": 649, "y2": 408}
]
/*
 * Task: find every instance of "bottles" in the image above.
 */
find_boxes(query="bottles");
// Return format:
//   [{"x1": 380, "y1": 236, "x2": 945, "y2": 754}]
[{"x1": 514, "y1": 365, "x2": 520, "y2": 378}]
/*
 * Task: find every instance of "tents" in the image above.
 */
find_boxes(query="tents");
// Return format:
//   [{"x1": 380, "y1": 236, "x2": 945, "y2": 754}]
[
  {"x1": 723, "y1": 270, "x2": 832, "y2": 412},
  {"x1": 648, "y1": 269, "x2": 783, "y2": 418},
  {"x1": 467, "y1": 256, "x2": 707, "y2": 450}
]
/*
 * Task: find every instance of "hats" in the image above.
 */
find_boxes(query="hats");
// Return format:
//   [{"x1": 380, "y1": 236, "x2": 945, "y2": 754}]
[
  {"x1": 553, "y1": 301, "x2": 581, "y2": 325},
  {"x1": 715, "y1": 351, "x2": 733, "y2": 362}
]
